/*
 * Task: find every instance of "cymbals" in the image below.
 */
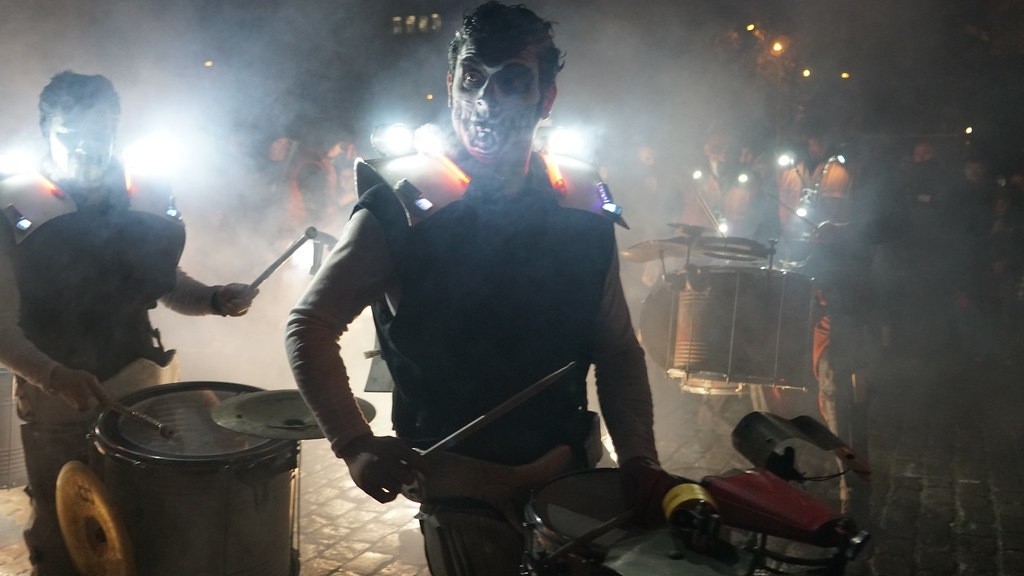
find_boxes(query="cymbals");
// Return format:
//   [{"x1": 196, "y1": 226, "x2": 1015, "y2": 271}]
[
  {"x1": 54, "y1": 458, "x2": 136, "y2": 575},
  {"x1": 621, "y1": 237, "x2": 682, "y2": 264},
  {"x1": 667, "y1": 222, "x2": 716, "y2": 234},
  {"x1": 210, "y1": 388, "x2": 377, "y2": 441},
  {"x1": 604, "y1": 525, "x2": 754, "y2": 576}
]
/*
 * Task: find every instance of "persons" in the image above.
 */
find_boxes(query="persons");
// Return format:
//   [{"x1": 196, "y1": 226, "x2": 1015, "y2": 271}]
[
  {"x1": 0, "y1": 70, "x2": 260, "y2": 576},
  {"x1": 283, "y1": 0, "x2": 670, "y2": 576},
  {"x1": 267, "y1": 98, "x2": 1024, "y2": 560}
]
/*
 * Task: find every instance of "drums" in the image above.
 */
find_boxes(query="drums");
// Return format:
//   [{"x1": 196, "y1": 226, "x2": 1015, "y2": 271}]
[
  {"x1": 521, "y1": 466, "x2": 725, "y2": 575},
  {"x1": 680, "y1": 377, "x2": 746, "y2": 397},
  {"x1": 91, "y1": 378, "x2": 301, "y2": 576},
  {"x1": 672, "y1": 263, "x2": 822, "y2": 392},
  {"x1": 641, "y1": 272, "x2": 680, "y2": 368}
]
